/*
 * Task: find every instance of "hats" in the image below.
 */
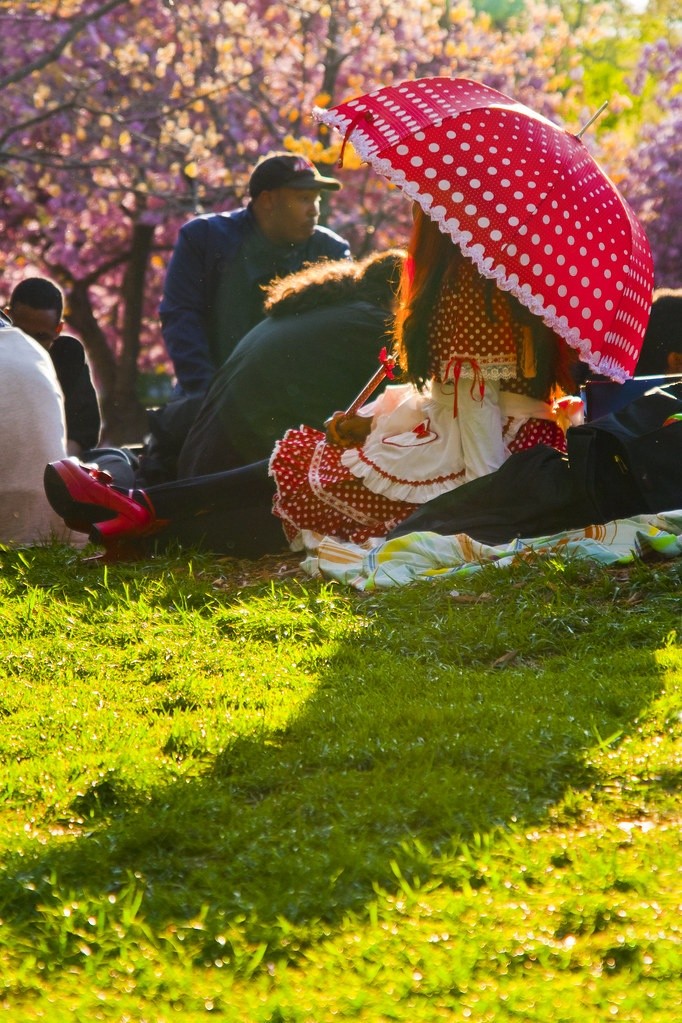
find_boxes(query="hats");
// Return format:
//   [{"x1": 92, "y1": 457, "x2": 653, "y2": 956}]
[{"x1": 249, "y1": 154, "x2": 342, "y2": 200}]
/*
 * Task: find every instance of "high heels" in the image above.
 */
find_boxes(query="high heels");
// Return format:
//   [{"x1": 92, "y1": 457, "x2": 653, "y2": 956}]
[
  {"x1": 44, "y1": 459, "x2": 153, "y2": 544},
  {"x1": 44, "y1": 459, "x2": 171, "y2": 566}
]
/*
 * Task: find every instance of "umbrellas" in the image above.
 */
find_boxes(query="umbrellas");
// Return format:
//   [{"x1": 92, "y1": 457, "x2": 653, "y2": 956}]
[{"x1": 311, "y1": 76, "x2": 655, "y2": 449}]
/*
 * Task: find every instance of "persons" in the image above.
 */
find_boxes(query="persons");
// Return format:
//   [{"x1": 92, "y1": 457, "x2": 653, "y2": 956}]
[
  {"x1": 631, "y1": 287, "x2": 682, "y2": 376},
  {"x1": 3, "y1": 277, "x2": 102, "y2": 456},
  {"x1": 141, "y1": 153, "x2": 351, "y2": 479},
  {"x1": 42, "y1": 201, "x2": 582, "y2": 560},
  {"x1": 0, "y1": 308, "x2": 89, "y2": 545}
]
